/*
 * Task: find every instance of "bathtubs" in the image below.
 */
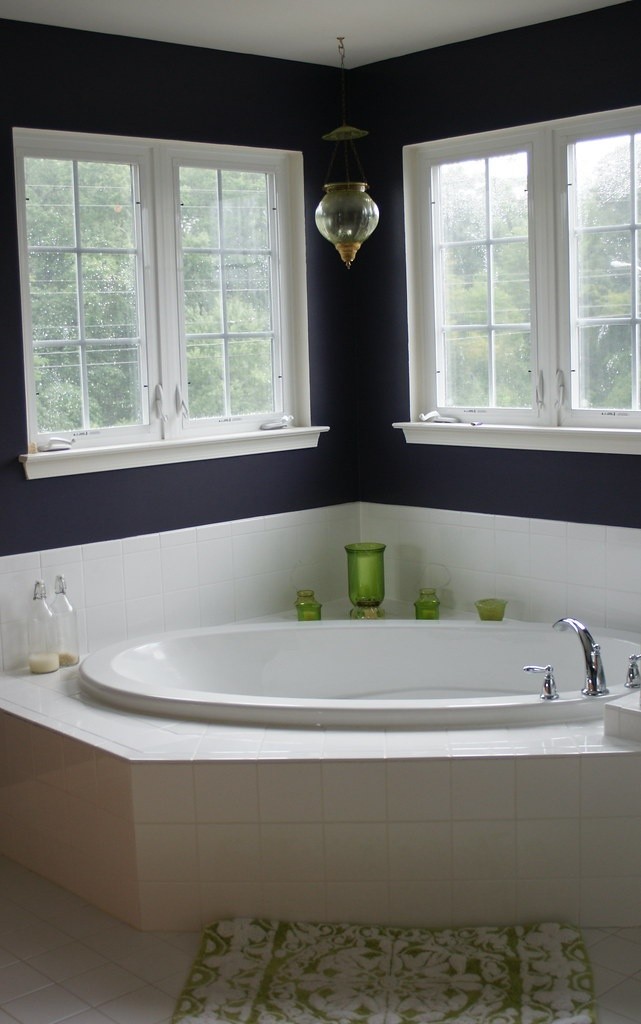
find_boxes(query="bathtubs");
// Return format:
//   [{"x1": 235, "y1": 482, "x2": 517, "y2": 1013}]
[{"x1": 79, "y1": 620, "x2": 641, "y2": 726}]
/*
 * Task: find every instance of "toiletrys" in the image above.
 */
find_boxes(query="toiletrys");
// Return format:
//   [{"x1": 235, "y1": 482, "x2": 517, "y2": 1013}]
[
  {"x1": 48, "y1": 574, "x2": 80, "y2": 668},
  {"x1": 27, "y1": 580, "x2": 59, "y2": 674}
]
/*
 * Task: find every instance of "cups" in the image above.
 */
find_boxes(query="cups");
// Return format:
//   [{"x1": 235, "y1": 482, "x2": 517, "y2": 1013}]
[
  {"x1": 475, "y1": 598, "x2": 508, "y2": 621},
  {"x1": 345, "y1": 543, "x2": 387, "y2": 620}
]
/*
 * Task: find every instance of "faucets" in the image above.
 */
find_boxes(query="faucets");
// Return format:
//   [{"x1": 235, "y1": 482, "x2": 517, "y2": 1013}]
[{"x1": 552, "y1": 617, "x2": 610, "y2": 697}]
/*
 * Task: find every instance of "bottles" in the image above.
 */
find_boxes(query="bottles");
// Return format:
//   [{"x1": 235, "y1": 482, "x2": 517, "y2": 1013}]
[
  {"x1": 26, "y1": 583, "x2": 60, "y2": 673},
  {"x1": 295, "y1": 590, "x2": 322, "y2": 621},
  {"x1": 53, "y1": 575, "x2": 78, "y2": 666},
  {"x1": 413, "y1": 589, "x2": 439, "y2": 620}
]
